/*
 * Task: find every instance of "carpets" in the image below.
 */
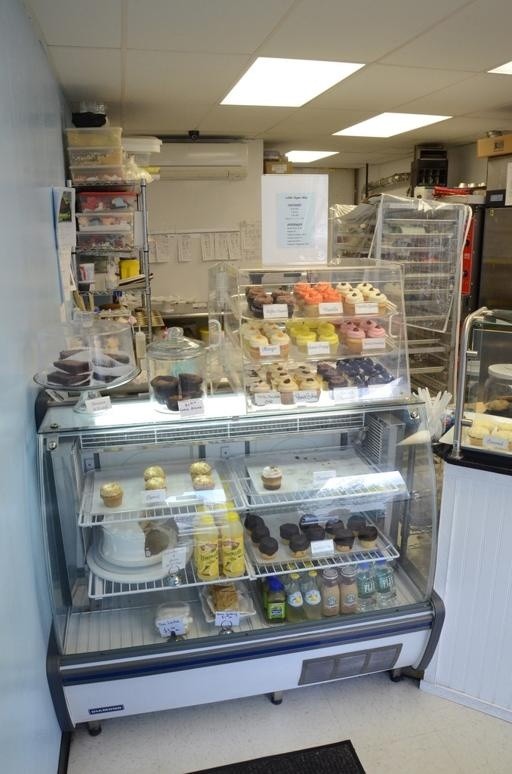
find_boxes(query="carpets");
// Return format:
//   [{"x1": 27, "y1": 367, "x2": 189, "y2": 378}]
[{"x1": 180, "y1": 739, "x2": 367, "y2": 774}]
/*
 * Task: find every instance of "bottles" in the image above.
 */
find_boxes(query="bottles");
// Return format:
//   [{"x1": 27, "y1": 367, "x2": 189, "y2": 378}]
[
  {"x1": 144, "y1": 327, "x2": 209, "y2": 414},
  {"x1": 262, "y1": 553, "x2": 397, "y2": 624},
  {"x1": 192, "y1": 503, "x2": 246, "y2": 580}
]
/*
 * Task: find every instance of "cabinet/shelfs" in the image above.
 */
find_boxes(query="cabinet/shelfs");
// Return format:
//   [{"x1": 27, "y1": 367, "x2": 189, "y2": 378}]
[{"x1": 36, "y1": 177, "x2": 512, "y2": 738}]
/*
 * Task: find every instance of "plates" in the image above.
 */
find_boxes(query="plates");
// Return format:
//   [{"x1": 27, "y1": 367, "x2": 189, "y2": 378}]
[{"x1": 198, "y1": 582, "x2": 256, "y2": 625}]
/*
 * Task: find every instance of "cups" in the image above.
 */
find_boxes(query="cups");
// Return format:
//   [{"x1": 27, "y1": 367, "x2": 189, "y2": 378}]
[{"x1": 78, "y1": 264, "x2": 95, "y2": 282}]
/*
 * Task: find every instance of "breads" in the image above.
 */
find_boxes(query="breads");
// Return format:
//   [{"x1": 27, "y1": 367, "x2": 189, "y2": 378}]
[
  {"x1": 244, "y1": 514, "x2": 377, "y2": 559},
  {"x1": 47, "y1": 349, "x2": 130, "y2": 391},
  {"x1": 150, "y1": 374, "x2": 204, "y2": 412}
]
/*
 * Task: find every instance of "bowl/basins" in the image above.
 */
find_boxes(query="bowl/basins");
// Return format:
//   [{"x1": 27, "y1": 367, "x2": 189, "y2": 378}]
[{"x1": 70, "y1": 111, "x2": 107, "y2": 128}]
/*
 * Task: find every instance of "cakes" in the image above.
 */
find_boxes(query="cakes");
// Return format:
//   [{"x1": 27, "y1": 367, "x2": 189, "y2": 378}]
[
  {"x1": 193, "y1": 474, "x2": 215, "y2": 491},
  {"x1": 143, "y1": 465, "x2": 165, "y2": 479},
  {"x1": 144, "y1": 477, "x2": 167, "y2": 491},
  {"x1": 242, "y1": 281, "x2": 395, "y2": 399},
  {"x1": 190, "y1": 461, "x2": 211, "y2": 479},
  {"x1": 99, "y1": 482, "x2": 123, "y2": 507},
  {"x1": 261, "y1": 465, "x2": 283, "y2": 490},
  {"x1": 100, "y1": 507, "x2": 179, "y2": 568},
  {"x1": 468, "y1": 420, "x2": 512, "y2": 451}
]
